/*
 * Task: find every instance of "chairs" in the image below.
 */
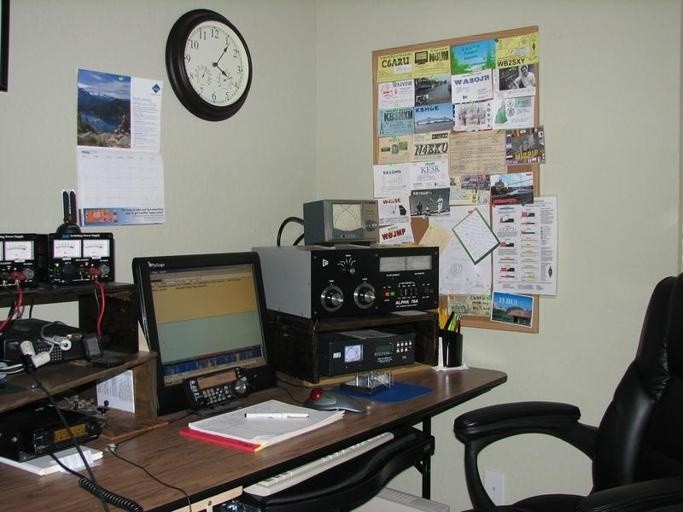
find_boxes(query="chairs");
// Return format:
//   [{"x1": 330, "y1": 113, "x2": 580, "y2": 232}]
[{"x1": 453, "y1": 273, "x2": 683, "y2": 512}]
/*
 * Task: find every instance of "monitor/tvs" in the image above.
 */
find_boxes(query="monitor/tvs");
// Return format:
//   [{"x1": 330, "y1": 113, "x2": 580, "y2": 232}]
[{"x1": 131, "y1": 251, "x2": 278, "y2": 418}]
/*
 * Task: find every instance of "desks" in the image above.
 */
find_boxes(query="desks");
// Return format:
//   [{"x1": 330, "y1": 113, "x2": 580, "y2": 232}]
[{"x1": 1, "y1": 351, "x2": 507, "y2": 511}]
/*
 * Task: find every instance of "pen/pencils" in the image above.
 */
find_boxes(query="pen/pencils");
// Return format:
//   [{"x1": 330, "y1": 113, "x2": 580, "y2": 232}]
[
  {"x1": 244, "y1": 412, "x2": 309, "y2": 419},
  {"x1": 444, "y1": 311, "x2": 460, "y2": 334}
]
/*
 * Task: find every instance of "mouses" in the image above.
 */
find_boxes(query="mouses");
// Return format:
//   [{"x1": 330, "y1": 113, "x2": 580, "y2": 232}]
[{"x1": 302, "y1": 391, "x2": 365, "y2": 414}]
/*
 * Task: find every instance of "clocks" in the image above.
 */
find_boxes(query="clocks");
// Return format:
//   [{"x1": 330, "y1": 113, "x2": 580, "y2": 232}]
[{"x1": 162, "y1": 7, "x2": 253, "y2": 122}]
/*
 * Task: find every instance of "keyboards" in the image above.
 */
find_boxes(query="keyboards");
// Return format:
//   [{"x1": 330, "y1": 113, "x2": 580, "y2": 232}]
[{"x1": 242, "y1": 432, "x2": 395, "y2": 497}]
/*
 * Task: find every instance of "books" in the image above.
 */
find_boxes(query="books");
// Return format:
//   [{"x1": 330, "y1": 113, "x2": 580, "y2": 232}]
[
  {"x1": 0, "y1": 441, "x2": 104, "y2": 476},
  {"x1": 176, "y1": 398, "x2": 348, "y2": 455}
]
